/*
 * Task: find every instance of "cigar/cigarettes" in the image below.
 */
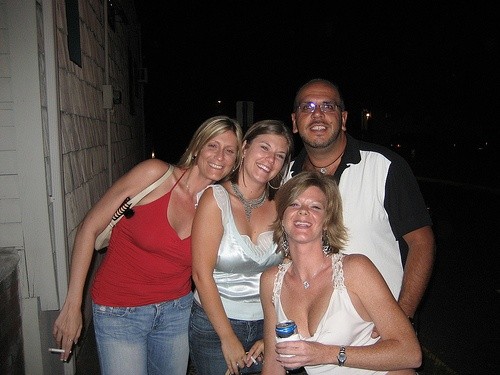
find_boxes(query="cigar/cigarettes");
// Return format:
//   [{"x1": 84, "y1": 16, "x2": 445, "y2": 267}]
[
  {"x1": 251, "y1": 356, "x2": 258, "y2": 366},
  {"x1": 48, "y1": 348, "x2": 71, "y2": 353}
]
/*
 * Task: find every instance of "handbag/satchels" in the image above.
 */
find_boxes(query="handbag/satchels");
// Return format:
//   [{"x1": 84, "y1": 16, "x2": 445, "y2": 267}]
[{"x1": 94, "y1": 163, "x2": 176, "y2": 251}]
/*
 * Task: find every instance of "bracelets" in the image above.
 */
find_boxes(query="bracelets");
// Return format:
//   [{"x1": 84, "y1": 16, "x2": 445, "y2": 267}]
[{"x1": 408, "y1": 316, "x2": 415, "y2": 326}]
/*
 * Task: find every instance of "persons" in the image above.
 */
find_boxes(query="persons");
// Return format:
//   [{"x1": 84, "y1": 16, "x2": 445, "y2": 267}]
[
  {"x1": 53, "y1": 117, "x2": 239, "y2": 375},
  {"x1": 188, "y1": 121, "x2": 294, "y2": 375},
  {"x1": 260, "y1": 171, "x2": 423, "y2": 375},
  {"x1": 290, "y1": 76, "x2": 436, "y2": 346}
]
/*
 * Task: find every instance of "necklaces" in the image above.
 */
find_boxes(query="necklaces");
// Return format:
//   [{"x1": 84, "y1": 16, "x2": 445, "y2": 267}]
[
  {"x1": 231, "y1": 182, "x2": 269, "y2": 220},
  {"x1": 294, "y1": 270, "x2": 317, "y2": 289},
  {"x1": 308, "y1": 151, "x2": 345, "y2": 175}
]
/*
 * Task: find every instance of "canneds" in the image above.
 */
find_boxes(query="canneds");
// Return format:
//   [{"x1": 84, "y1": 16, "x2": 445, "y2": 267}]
[{"x1": 275, "y1": 320, "x2": 298, "y2": 338}]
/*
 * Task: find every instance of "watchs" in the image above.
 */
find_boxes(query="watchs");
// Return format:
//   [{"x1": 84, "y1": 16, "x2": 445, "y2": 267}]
[{"x1": 337, "y1": 345, "x2": 347, "y2": 366}]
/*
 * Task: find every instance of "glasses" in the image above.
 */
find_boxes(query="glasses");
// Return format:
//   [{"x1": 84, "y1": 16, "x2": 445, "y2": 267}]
[{"x1": 296, "y1": 101, "x2": 342, "y2": 113}]
